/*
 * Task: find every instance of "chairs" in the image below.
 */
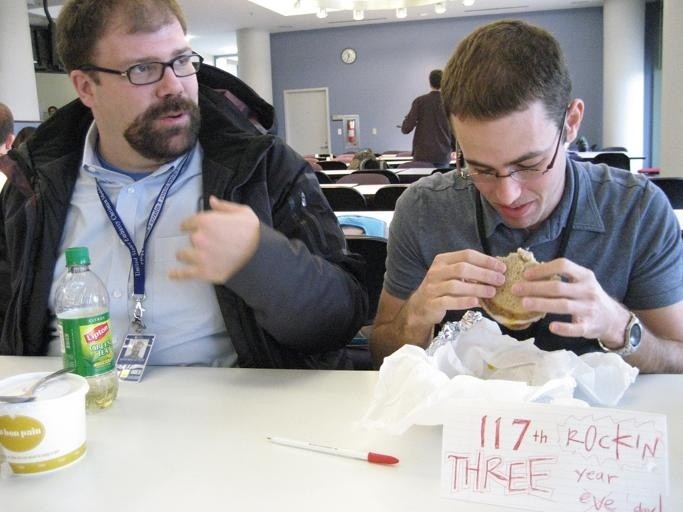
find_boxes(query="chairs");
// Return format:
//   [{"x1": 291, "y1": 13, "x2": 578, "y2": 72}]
[
  {"x1": 283, "y1": 144, "x2": 458, "y2": 266},
  {"x1": 566, "y1": 144, "x2": 680, "y2": 227}
]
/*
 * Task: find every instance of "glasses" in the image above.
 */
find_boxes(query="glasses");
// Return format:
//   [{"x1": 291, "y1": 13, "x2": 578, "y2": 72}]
[
  {"x1": 76, "y1": 50, "x2": 204, "y2": 87},
  {"x1": 454, "y1": 109, "x2": 567, "y2": 185}
]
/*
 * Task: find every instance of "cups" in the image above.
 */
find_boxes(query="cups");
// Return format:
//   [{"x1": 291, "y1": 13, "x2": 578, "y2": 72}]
[{"x1": 0, "y1": 372, "x2": 91, "y2": 477}]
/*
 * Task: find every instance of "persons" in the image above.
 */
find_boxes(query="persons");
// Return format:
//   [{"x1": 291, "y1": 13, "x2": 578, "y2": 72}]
[
  {"x1": 1, "y1": 0, "x2": 370, "y2": 368},
  {"x1": 0, "y1": 102, "x2": 16, "y2": 191},
  {"x1": 400, "y1": 68, "x2": 455, "y2": 168},
  {"x1": 48, "y1": 106, "x2": 57, "y2": 117},
  {"x1": 369, "y1": 17, "x2": 682, "y2": 375}
]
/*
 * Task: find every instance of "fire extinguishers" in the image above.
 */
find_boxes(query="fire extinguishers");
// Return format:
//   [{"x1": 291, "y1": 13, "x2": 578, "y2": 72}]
[{"x1": 347, "y1": 120, "x2": 356, "y2": 143}]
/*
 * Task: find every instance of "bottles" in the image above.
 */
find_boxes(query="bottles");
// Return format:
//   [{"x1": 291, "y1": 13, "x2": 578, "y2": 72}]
[{"x1": 52, "y1": 246, "x2": 118, "y2": 409}]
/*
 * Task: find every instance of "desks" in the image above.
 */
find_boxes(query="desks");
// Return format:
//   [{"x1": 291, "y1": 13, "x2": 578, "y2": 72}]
[{"x1": 0, "y1": 352, "x2": 682, "y2": 512}]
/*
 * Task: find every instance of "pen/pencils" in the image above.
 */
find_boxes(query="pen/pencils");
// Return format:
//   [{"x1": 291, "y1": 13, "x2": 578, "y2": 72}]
[{"x1": 267, "y1": 438, "x2": 399, "y2": 464}]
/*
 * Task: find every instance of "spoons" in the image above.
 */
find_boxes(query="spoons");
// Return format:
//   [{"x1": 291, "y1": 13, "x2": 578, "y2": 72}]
[{"x1": 0, "y1": 363, "x2": 73, "y2": 405}]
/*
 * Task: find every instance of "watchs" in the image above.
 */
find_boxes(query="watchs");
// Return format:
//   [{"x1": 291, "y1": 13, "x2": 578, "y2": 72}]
[{"x1": 598, "y1": 310, "x2": 645, "y2": 355}]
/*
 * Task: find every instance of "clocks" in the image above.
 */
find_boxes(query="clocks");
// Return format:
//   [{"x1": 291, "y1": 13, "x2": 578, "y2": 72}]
[{"x1": 340, "y1": 48, "x2": 357, "y2": 66}]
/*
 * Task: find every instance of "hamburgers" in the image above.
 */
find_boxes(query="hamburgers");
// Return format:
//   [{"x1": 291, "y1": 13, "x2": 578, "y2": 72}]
[{"x1": 479, "y1": 249, "x2": 562, "y2": 332}]
